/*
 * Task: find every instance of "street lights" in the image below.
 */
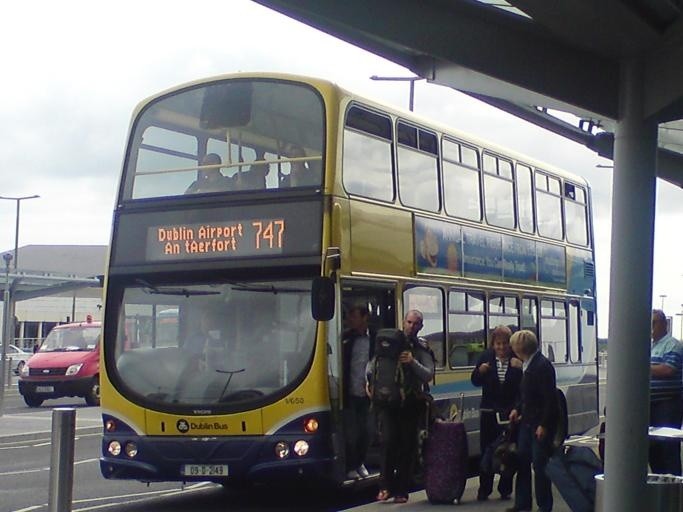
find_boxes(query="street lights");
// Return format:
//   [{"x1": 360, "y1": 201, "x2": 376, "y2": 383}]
[
  {"x1": 0, "y1": 194, "x2": 39, "y2": 274},
  {"x1": 0, "y1": 253, "x2": 12, "y2": 416},
  {"x1": 370, "y1": 74, "x2": 424, "y2": 112}
]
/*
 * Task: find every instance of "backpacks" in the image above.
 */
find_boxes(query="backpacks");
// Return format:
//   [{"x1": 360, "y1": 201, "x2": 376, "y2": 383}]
[{"x1": 367, "y1": 328, "x2": 416, "y2": 409}]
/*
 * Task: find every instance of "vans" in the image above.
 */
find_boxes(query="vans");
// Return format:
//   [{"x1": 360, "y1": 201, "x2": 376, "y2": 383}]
[{"x1": 19, "y1": 318, "x2": 139, "y2": 406}]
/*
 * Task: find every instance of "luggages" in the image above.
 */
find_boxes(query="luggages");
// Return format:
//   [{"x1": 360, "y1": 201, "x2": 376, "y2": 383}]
[
  {"x1": 422, "y1": 393, "x2": 468, "y2": 504},
  {"x1": 534, "y1": 434, "x2": 604, "y2": 512}
]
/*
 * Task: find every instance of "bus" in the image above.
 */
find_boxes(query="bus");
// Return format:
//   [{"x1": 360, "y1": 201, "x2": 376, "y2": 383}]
[{"x1": 98, "y1": 71, "x2": 598, "y2": 492}]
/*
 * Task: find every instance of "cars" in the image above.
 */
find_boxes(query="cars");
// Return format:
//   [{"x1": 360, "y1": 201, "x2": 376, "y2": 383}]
[{"x1": 10, "y1": 344, "x2": 35, "y2": 373}]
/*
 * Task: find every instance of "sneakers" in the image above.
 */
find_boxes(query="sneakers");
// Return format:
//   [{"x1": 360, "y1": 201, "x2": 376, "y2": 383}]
[{"x1": 346, "y1": 464, "x2": 369, "y2": 479}]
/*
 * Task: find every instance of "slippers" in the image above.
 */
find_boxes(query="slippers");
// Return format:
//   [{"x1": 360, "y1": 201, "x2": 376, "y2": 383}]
[{"x1": 377, "y1": 488, "x2": 408, "y2": 503}]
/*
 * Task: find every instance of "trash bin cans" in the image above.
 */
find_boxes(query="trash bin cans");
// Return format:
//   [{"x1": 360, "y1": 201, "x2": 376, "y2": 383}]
[{"x1": 594, "y1": 473, "x2": 683, "y2": 512}]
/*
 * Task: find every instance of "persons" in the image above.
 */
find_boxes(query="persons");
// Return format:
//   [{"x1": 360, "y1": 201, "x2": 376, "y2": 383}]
[
  {"x1": 183, "y1": 145, "x2": 321, "y2": 196},
  {"x1": 182, "y1": 299, "x2": 436, "y2": 503},
  {"x1": 470, "y1": 326, "x2": 558, "y2": 512},
  {"x1": 649, "y1": 309, "x2": 682, "y2": 475}
]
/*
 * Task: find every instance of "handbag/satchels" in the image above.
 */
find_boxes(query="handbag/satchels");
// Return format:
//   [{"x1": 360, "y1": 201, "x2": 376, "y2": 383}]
[
  {"x1": 418, "y1": 392, "x2": 433, "y2": 431},
  {"x1": 495, "y1": 429, "x2": 521, "y2": 464}
]
